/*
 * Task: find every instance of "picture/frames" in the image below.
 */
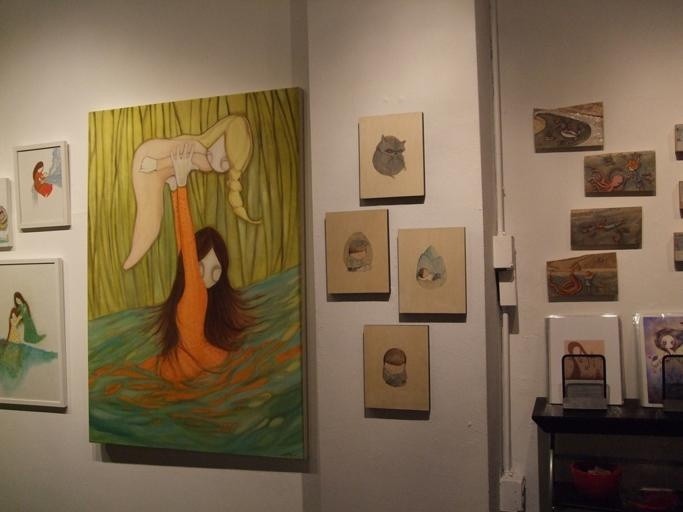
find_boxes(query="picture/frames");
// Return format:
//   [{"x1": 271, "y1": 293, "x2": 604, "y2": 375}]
[
  {"x1": 546, "y1": 311, "x2": 681, "y2": 409},
  {"x1": 0, "y1": 139, "x2": 73, "y2": 412}
]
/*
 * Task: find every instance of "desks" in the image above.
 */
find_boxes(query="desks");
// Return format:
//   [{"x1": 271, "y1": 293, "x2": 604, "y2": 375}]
[{"x1": 532, "y1": 396, "x2": 683, "y2": 512}]
